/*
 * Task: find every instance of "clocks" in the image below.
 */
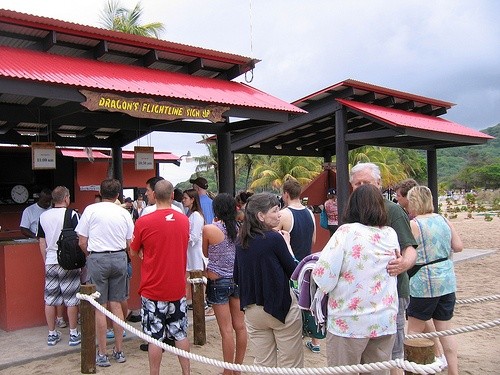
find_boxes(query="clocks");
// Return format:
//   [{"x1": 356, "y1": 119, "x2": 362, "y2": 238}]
[{"x1": 11, "y1": 185, "x2": 29, "y2": 204}]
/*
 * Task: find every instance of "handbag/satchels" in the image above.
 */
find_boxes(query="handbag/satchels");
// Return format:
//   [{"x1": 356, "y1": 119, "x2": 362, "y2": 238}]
[
  {"x1": 57, "y1": 209, "x2": 85, "y2": 270},
  {"x1": 320, "y1": 206, "x2": 328, "y2": 229},
  {"x1": 407, "y1": 265, "x2": 422, "y2": 280},
  {"x1": 289, "y1": 260, "x2": 326, "y2": 339}
]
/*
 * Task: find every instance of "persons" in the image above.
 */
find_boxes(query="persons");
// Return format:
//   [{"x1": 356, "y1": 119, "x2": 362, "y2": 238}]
[
  {"x1": 20, "y1": 185, "x2": 54, "y2": 239},
  {"x1": 275, "y1": 180, "x2": 322, "y2": 354},
  {"x1": 90, "y1": 174, "x2": 255, "y2": 353},
  {"x1": 35, "y1": 185, "x2": 83, "y2": 345},
  {"x1": 201, "y1": 193, "x2": 248, "y2": 375},
  {"x1": 129, "y1": 180, "x2": 193, "y2": 375},
  {"x1": 350, "y1": 163, "x2": 418, "y2": 375},
  {"x1": 324, "y1": 190, "x2": 339, "y2": 238},
  {"x1": 315, "y1": 186, "x2": 401, "y2": 375},
  {"x1": 393, "y1": 177, "x2": 463, "y2": 374},
  {"x1": 56, "y1": 302, "x2": 83, "y2": 328},
  {"x1": 235, "y1": 192, "x2": 304, "y2": 369},
  {"x1": 76, "y1": 180, "x2": 136, "y2": 367}
]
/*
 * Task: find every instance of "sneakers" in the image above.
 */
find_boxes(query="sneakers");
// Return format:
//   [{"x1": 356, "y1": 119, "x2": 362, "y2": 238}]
[
  {"x1": 47, "y1": 331, "x2": 62, "y2": 346},
  {"x1": 306, "y1": 340, "x2": 321, "y2": 353},
  {"x1": 108, "y1": 347, "x2": 126, "y2": 362},
  {"x1": 69, "y1": 334, "x2": 81, "y2": 345},
  {"x1": 96, "y1": 350, "x2": 110, "y2": 367},
  {"x1": 55, "y1": 318, "x2": 66, "y2": 327}
]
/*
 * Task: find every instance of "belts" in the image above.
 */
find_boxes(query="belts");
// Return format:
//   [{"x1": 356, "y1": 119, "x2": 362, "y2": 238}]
[{"x1": 89, "y1": 249, "x2": 125, "y2": 254}]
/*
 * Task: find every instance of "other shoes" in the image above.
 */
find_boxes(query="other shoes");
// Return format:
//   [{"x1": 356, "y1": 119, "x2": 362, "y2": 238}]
[
  {"x1": 107, "y1": 328, "x2": 126, "y2": 337},
  {"x1": 188, "y1": 301, "x2": 208, "y2": 309},
  {"x1": 140, "y1": 344, "x2": 164, "y2": 352},
  {"x1": 206, "y1": 299, "x2": 212, "y2": 307}
]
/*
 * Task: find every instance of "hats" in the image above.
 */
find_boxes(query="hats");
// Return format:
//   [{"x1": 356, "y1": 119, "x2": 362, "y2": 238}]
[
  {"x1": 189, "y1": 177, "x2": 208, "y2": 189},
  {"x1": 328, "y1": 188, "x2": 336, "y2": 194},
  {"x1": 124, "y1": 197, "x2": 134, "y2": 203}
]
